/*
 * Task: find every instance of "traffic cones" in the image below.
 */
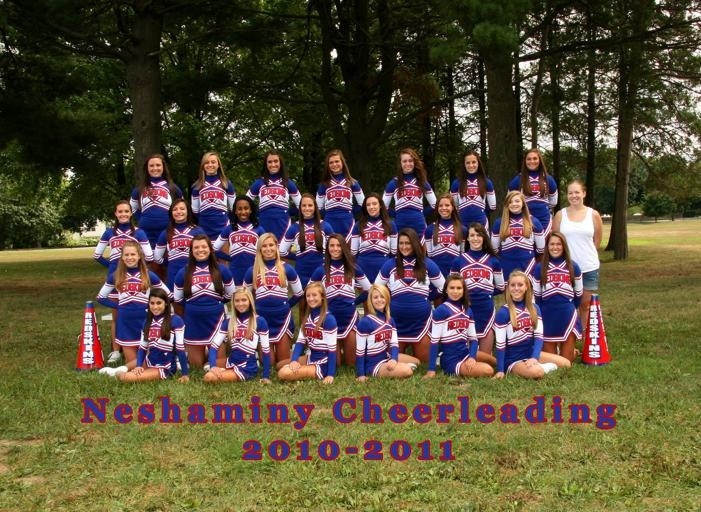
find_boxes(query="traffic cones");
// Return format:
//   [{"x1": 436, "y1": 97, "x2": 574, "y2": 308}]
[
  {"x1": 76, "y1": 301, "x2": 105, "y2": 370},
  {"x1": 580, "y1": 292, "x2": 611, "y2": 364}
]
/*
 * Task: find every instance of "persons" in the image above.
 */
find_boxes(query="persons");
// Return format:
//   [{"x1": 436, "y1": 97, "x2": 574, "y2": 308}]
[
  {"x1": 493, "y1": 270, "x2": 572, "y2": 380},
  {"x1": 421, "y1": 271, "x2": 496, "y2": 381},
  {"x1": 354, "y1": 284, "x2": 420, "y2": 383},
  {"x1": 552, "y1": 178, "x2": 603, "y2": 339},
  {"x1": 276, "y1": 281, "x2": 338, "y2": 385},
  {"x1": 203, "y1": 286, "x2": 272, "y2": 385},
  {"x1": 98, "y1": 288, "x2": 190, "y2": 384},
  {"x1": 93, "y1": 148, "x2": 583, "y2": 368}
]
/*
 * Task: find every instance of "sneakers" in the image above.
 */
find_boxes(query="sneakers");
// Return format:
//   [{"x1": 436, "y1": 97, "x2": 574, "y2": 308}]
[
  {"x1": 107, "y1": 352, "x2": 120, "y2": 363},
  {"x1": 542, "y1": 363, "x2": 558, "y2": 374},
  {"x1": 99, "y1": 367, "x2": 117, "y2": 377},
  {"x1": 204, "y1": 364, "x2": 210, "y2": 371},
  {"x1": 176, "y1": 362, "x2": 190, "y2": 370}
]
